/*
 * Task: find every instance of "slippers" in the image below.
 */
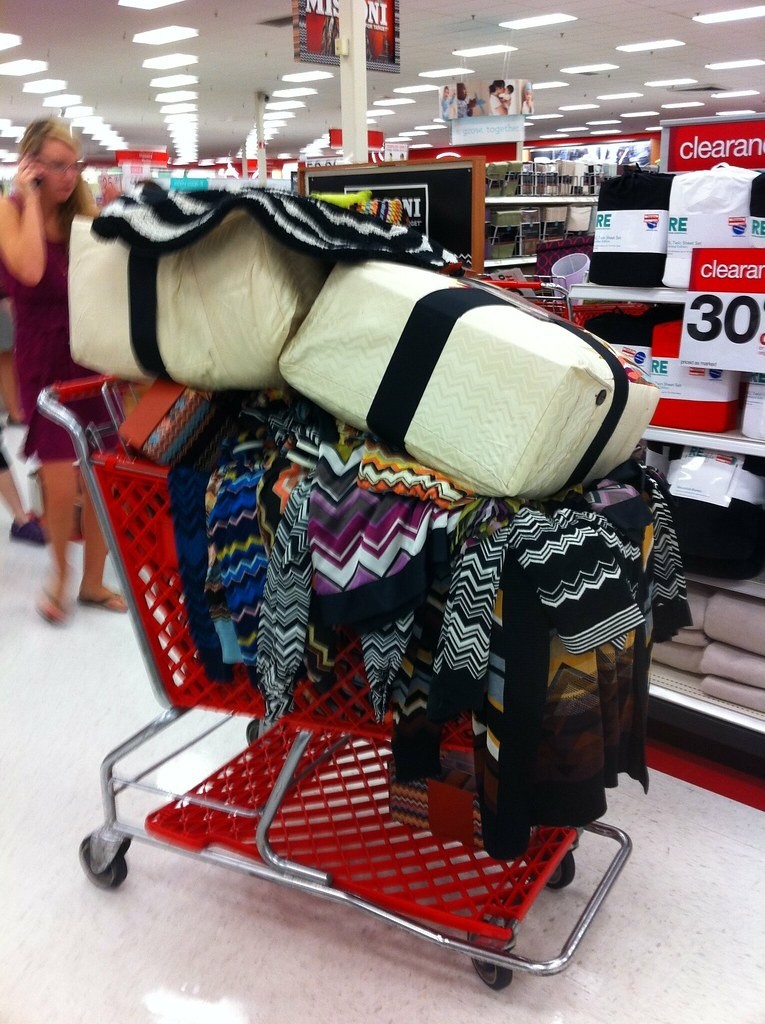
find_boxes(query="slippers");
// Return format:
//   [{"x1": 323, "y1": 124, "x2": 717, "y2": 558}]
[
  {"x1": 34, "y1": 586, "x2": 69, "y2": 625},
  {"x1": 78, "y1": 589, "x2": 128, "y2": 613}
]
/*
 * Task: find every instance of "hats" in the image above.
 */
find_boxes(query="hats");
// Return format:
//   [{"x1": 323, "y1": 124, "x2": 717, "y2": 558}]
[{"x1": 525, "y1": 82, "x2": 531, "y2": 90}]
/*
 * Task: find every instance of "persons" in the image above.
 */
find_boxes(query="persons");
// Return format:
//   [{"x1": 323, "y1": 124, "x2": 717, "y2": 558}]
[
  {"x1": 440, "y1": 80, "x2": 533, "y2": 121},
  {"x1": 0, "y1": 116, "x2": 128, "y2": 623}
]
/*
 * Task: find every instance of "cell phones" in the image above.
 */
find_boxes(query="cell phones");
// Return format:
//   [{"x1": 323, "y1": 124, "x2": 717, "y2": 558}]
[{"x1": 32, "y1": 176, "x2": 44, "y2": 190}]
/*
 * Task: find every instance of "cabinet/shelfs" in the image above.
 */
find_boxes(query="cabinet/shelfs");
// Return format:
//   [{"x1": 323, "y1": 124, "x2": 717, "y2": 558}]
[
  {"x1": 566, "y1": 279, "x2": 765, "y2": 735},
  {"x1": 484, "y1": 195, "x2": 599, "y2": 270}
]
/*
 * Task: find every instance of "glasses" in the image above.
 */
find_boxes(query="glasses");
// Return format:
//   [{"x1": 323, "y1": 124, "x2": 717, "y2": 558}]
[{"x1": 37, "y1": 155, "x2": 89, "y2": 176}]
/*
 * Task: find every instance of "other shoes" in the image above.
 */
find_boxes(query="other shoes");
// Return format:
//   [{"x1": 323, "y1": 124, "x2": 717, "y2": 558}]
[{"x1": 11, "y1": 518, "x2": 46, "y2": 545}]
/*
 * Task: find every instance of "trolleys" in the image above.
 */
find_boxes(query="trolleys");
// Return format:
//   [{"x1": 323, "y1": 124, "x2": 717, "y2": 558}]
[{"x1": 35, "y1": 376, "x2": 632, "y2": 993}]
